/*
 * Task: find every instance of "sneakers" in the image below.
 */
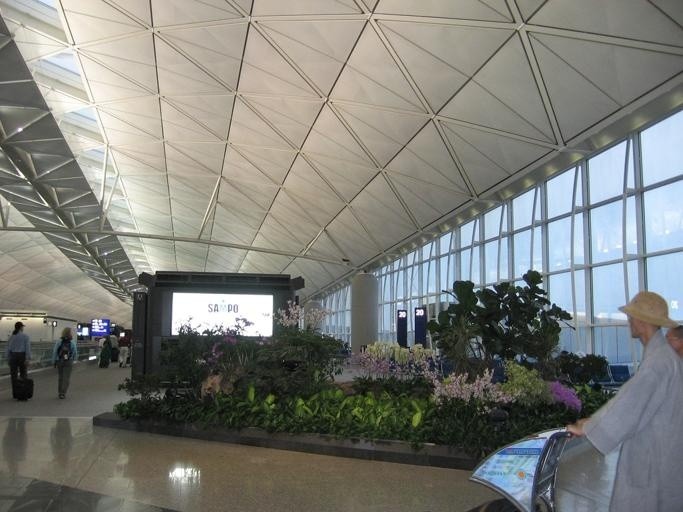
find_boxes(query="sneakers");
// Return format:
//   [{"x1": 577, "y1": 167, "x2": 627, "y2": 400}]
[
  {"x1": 119, "y1": 362, "x2": 128, "y2": 368},
  {"x1": 58, "y1": 393, "x2": 65, "y2": 399}
]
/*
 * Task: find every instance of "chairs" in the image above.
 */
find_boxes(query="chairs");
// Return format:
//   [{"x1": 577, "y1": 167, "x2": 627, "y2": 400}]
[{"x1": 568, "y1": 364, "x2": 630, "y2": 394}]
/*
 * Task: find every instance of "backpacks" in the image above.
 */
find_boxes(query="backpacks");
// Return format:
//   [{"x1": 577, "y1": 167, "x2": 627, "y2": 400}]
[{"x1": 57, "y1": 337, "x2": 72, "y2": 361}]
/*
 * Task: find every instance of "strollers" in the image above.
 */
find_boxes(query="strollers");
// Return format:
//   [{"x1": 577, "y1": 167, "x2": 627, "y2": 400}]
[{"x1": 467, "y1": 427, "x2": 572, "y2": 512}]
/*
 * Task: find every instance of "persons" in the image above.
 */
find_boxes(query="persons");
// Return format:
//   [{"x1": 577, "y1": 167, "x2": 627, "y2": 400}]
[
  {"x1": 96, "y1": 326, "x2": 132, "y2": 368},
  {"x1": 566, "y1": 291, "x2": 682, "y2": 511},
  {"x1": 664, "y1": 325, "x2": 682, "y2": 358},
  {"x1": 51, "y1": 326, "x2": 77, "y2": 398},
  {"x1": 4, "y1": 322, "x2": 33, "y2": 401}
]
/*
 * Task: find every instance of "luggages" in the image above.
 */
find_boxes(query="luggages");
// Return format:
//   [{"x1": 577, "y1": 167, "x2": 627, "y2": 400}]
[{"x1": 13, "y1": 368, "x2": 34, "y2": 402}]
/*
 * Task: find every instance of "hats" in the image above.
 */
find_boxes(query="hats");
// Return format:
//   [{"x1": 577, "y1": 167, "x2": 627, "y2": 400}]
[
  {"x1": 14, "y1": 322, "x2": 26, "y2": 329},
  {"x1": 617, "y1": 291, "x2": 679, "y2": 329}
]
